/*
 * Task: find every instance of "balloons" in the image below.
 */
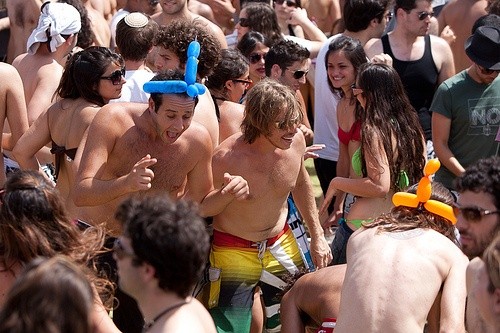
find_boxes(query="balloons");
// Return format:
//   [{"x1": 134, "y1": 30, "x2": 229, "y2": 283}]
[
  {"x1": 392, "y1": 158, "x2": 458, "y2": 225},
  {"x1": 143, "y1": 39, "x2": 206, "y2": 97}
]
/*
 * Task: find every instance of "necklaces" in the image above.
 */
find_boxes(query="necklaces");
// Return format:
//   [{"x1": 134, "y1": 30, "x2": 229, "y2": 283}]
[{"x1": 145, "y1": 302, "x2": 191, "y2": 330}]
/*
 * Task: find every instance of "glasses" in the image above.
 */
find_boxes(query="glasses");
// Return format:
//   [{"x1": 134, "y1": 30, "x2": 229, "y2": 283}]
[
  {"x1": 101, "y1": 66, "x2": 126, "y2": 85},
  {"x1": 286, "y1": 68, "x2": 309, "y2": 79},
  {"x1": 274, "y1": 0, "x2": 298, "y2": 8},
  {"x1": 224, "y1": 79, "x2": 253, "y2": 90},
  {"x1": 250, "y1": 53, "x2": 267, "y2": 64},
  {"x1": 113, "y1": 239, "x2": 136, "y2": 262},
  {"x1": 239, "y1": 18, "x2": 250, "y2": 27},
  {"x1": 351, "y1": 81, "x2": 363, "y2": 97},
  {"x1": 148, "y1": 0, "x2": 159, "y2": 7},
  {"x1": 272, "y1": 114, "x2": 300, "y2": 129},
  {"x1": 451, "y1": 202, "x2": 500, "y2": 222},
  {"x1": 410, "y1": 11, "x2": 435, "y2": 21}
]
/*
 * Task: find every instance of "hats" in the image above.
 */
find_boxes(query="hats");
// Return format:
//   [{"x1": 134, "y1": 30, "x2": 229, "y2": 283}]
[{"x1": 463, "y1": 26, "x2": 500, "y2": 70}]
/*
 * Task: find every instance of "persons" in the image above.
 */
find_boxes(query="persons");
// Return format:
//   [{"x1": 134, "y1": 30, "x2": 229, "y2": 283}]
[{"x1": 0, "y1": 0, "x2": 500, "y2": 333}]
